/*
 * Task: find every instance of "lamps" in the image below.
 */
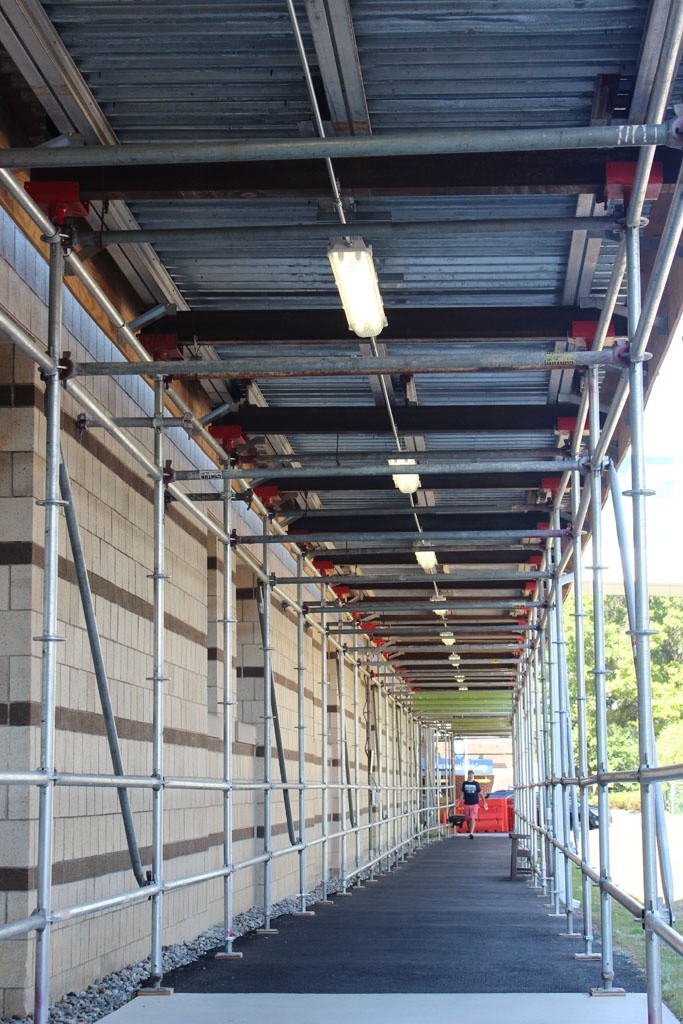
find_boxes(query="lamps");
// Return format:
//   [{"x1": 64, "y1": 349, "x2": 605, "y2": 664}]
[
  {"x1": 449, "y1": 654, "x2": 460, "y2": 667},
  {"x1": 429, "y1": 596, "x2": 447, "y2": 615},
  {"x1": 387, "y1": 458, "x2": 421, "y2": 494},
  {"x1": 413, "y1": 540, "x2": 436, "y2": 569},
  {"x1": 325, "y1": 236, "x2": 387, "y2": 338},
  {"x1": 441, "y1": 632, "x2": 456, "y2": 646}
]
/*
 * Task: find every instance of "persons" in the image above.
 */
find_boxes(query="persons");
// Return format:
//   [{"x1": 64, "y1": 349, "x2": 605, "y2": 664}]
[{"x1": 455, "y1": 770, "x2": 488, "y2": 840}]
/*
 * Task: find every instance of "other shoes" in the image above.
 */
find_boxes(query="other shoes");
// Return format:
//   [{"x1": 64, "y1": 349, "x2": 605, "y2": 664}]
[{"x1": 470, "y1": 835, "x2": 473, "y2": 839}]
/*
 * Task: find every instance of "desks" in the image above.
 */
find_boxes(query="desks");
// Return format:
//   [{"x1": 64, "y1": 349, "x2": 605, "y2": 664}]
[{"x1": 509, "y1": 833, "x2": 543, "y2": 881}]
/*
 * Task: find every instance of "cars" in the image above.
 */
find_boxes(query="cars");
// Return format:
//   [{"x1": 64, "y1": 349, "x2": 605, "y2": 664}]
[{"x1": 484, "y1": 790, "x2": 612, "y2": 830}]
